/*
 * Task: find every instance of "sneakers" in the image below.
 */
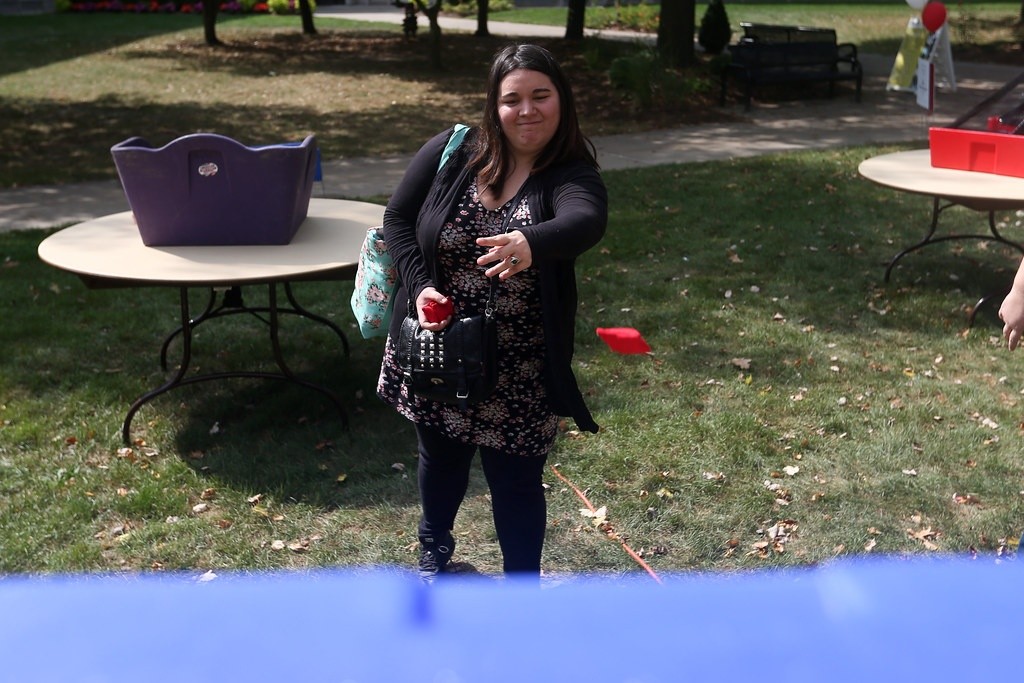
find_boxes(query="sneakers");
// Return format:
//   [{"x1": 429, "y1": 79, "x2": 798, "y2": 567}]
[{"x1": 417, "y1": 538, "x2": 456, "y2": 584}]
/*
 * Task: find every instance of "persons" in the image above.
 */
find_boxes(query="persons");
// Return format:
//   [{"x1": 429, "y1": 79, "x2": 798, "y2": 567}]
[
  {"x1": 376, "y1": 44, "x2": 608, "y2": 588},
  {"x1": 998, "y1": 257, "x2": 1024, "y2": 352}
]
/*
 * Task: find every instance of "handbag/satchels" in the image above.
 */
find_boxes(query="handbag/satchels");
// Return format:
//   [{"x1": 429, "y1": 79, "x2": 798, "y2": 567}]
[
  {"x1": 350, "y1": 123, "x2": 472, "y2": 340},
  {"x1": 395, "y1": 299, "x2": 498, "y2": 405}
]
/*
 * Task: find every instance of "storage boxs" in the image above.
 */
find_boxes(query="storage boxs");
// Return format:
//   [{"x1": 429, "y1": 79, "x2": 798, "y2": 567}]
[{"x1": 111, "y1": 134, "x2": 318, "y2": 247}]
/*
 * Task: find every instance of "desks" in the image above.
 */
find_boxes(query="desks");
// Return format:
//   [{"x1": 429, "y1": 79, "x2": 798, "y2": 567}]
[
  {"x1": 858, "y1": 149, "x2": 1024, "y2": 326},
  {"x1": 38, "y1": 196, "x2": 388, "y2": 445}
]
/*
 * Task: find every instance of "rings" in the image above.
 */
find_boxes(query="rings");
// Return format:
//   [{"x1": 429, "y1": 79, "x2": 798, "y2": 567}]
[{"x1": 511, "y1": 257, "x2": 519, "y2": 265}]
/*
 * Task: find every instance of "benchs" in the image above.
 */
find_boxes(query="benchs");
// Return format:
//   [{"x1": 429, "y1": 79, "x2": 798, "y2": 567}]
[{"x1": 719, "y1": 21, "x2": 863, "y2": 112}]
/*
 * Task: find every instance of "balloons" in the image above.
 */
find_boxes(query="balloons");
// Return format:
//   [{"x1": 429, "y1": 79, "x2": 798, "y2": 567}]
[{"x1": 906, "y1": 0, "x2": 947, "y2": 33}]
